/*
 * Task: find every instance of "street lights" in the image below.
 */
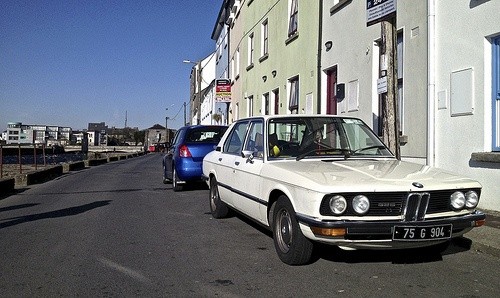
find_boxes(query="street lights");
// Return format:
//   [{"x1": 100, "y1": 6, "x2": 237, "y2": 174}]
[{"x1": 183, "y1": 60, "x2": 202, "y2": 124}]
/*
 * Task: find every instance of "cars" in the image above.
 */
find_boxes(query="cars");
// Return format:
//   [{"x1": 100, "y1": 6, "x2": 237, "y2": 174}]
[
  {"x1": 201, "y1": 115, "x2": 485, "y2": 267},
  {"x1": 162, "y1": 123, "x2": 242, "y2": 192}
]
